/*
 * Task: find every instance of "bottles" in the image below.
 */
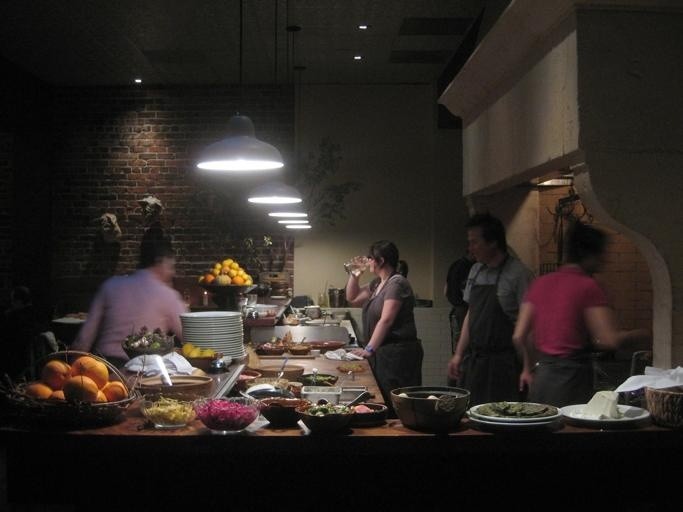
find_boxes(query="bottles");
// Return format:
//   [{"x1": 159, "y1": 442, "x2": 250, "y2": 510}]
[
  {"x1": 317, "y1": 288, "x2": 325, "y2": 308},
  {"x1": 239, "y1": 298, "x2": 258, "y2": 320}
]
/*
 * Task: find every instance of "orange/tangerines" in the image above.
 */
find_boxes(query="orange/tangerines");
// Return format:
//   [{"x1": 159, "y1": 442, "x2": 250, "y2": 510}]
[{"x1": 198, "y1": 258, "x2": 253, "y2": 285}]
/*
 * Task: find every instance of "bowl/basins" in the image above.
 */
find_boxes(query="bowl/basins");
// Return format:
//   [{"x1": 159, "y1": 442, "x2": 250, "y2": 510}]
[
  {"x1": 341, "y1": 402, "x2": 389, "y2": 428},
  {"x1": 295, "y1": 403, "x2": 357, "y2": 432},
  {"x1": 246, "y1": 388, "x2": 295, "y2": 401},
  {"x1": 256, "y1": 396, "x2": 312, "y2": 428},
  {"x1": 140, "y1": 398, "x2": 205, "y2": 429},
  {"x1": 303, "y1": 304, "x2": 318, "y2": 319},
  {"x1": 253, "y1": 339, "x2": 346, "y2": 357},
  {"x1": 646, "y1": 387, "x2": 683, "y2": 426},
  {"x1": 120, "y1": 335, "x2": 173, "y2": 361},
  {"x1": 391, "y1": 386, "x2": 468, "y2": 432},
  {"x1": 236, "y1": 363, "x2": 336, "y2": 385},
  {"x1": 194, "y1": 398, "x2": 262, "y2": 433},
  {"x1": 137, "y1": 376, "x2": 214, "y2": 399},
  {"x1": 300, "y1": 386, "x2": 342, "y2": 404}
]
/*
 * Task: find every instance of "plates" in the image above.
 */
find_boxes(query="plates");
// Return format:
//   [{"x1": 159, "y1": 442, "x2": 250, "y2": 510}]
[
  {"x1": 468, "y1": 402, "x2": 648, "y2": 426},
  {"x1": 269, "y1": 296, "x2": 285, "y2": 299},
  {"x1": 202, "y1": 285, "x2": 258, "y2": 294},
  {"x1": 178, "y1": 310, "x2": 246, "y2": 368}
]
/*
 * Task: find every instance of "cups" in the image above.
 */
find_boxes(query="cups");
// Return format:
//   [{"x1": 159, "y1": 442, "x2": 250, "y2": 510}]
[
  {"x1": 327, "y1": 287, "x2": 347, "y2": 308},
  {"x1": 343, "y1": 256, "x2": 370, "y2": 274},
  {"x1": 271, "y1": 281, "x2": 285, "y2": 296}
]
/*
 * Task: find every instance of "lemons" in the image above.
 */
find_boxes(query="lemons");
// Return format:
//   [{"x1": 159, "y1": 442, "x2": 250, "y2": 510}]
[{"x1": 182, "y1": 343, "x2": 214, "y2": 358}]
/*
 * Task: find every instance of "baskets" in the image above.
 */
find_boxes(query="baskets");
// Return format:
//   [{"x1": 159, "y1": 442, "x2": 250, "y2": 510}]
[
  {"x1": 5, "y1": 350, "x2": 136, "y2": 429},
  {"x1": 128, "y1": 373, "x2": 215, "y2": 401}
]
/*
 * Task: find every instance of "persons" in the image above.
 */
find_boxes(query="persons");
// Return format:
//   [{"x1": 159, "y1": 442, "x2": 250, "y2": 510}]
[
  {"x1": 71, "y1": 228, "x2": 191, "y2": 374},
  {"x1": 443, "y1": 246, "x2": 478, "y2": 389},
  {"x1": 446, "y1": 210, "x2": 536, "y2": 405},
  {"x1": 510, "y1": 223, "x2": 653, "y2": 407},
  {"x1": 343, "y1": 239, "x2": 424, "y2": 419},
  {"x1": 1, "y1": 285, "x2": 36, "y2": 382}
]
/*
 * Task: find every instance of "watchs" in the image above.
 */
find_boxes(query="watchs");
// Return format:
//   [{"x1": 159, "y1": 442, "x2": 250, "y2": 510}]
[{"x1": 365, "y1": 346, "x2": 375, "y2": 354}]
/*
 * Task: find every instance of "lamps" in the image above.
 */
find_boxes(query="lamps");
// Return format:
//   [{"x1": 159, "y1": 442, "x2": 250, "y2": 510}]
[{"x1": 186, "y1": 0, "x2": 318, "y2": 235}]
